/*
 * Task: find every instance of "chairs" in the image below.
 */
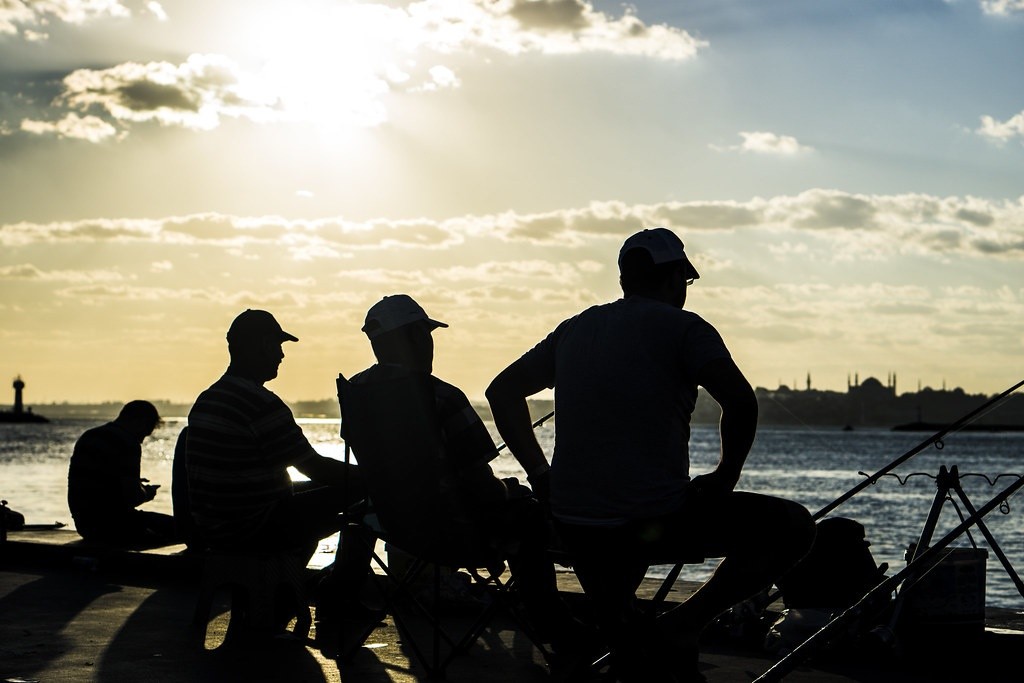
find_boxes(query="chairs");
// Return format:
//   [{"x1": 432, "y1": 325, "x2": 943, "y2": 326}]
[{"x1": 172, "y1": 372, "x2": 702, "y2": 683}]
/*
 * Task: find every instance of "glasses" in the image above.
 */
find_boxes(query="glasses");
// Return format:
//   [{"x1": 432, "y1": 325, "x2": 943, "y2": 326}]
[{"x1": 682, "y1": 272, "x2": 694, "y2": 285}]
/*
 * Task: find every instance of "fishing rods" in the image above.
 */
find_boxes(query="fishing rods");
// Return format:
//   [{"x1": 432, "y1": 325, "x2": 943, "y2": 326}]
[
  {"x1": 496, "y1": 409, "x2": 554, "y2": 451},
  {"x1": 751, "y1": 475, "x2": 1024, "y2": 683},
  {"x1": 590, "y1": 378, "x2": 1024, "y2": 671}
]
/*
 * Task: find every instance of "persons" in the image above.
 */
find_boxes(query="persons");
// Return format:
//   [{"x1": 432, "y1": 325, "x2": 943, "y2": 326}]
[
  {"x1": 342, "y1": 295, "x2": 556, "y2": 631},
  {"x1": 68, "y1": 401, "x2": 174, "y2": 543},
  {"x1": 185, "y1": 310, "x2": 386, "y2": 624},
  {"x1": 485, "y1": 228, "x2": 816, "y2": 664}
]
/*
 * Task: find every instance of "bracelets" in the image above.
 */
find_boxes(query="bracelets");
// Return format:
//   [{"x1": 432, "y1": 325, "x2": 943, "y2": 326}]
[{"x1": 527, "y1": 462, "x2": 548, "y2": 484}]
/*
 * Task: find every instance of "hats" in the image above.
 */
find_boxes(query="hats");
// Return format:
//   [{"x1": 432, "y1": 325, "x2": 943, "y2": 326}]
[
  {"x1": 228, "y1": 309, "x2": 299, "y2": 343},
  {"x1": 618, "y1": 228, "x2": 701, "y2": 279},
  {"x1": 361, "y1": 294, "x2": 448, "y2": 338}
]
